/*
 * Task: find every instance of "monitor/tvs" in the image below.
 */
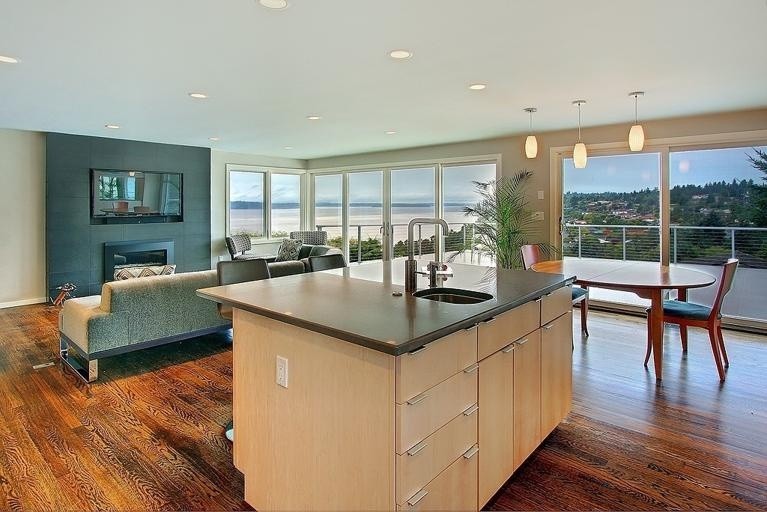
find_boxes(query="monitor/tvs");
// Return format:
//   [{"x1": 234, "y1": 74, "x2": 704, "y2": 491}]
[{"x1": 89, "y1": 167, "x2": 183, "y2": 225}]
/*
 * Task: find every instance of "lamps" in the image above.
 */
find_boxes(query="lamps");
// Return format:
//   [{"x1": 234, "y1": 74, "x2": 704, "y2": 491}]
[
  {"x1": 523, "y1": 106, "x2": 538, "y2": 160},
  {"x1": 572, "y1": 98, "x2": 588, "y2": 168},
  {"x1": 627, "y1": 91, "x2": 645, "y2": 153}
]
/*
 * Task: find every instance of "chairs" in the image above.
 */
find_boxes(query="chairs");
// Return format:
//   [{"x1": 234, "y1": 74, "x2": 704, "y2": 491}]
[
  {"x1": 520, "y1": 244, "x2": 590, "y2": 351},
  {"x1": 216, "y1": 258, "x2": 272, "y2": 443},
  {"x1": 112, "y1": 201, "x2": 129, "y2": 216},
  {"x1": 134, "y1": 206, "x2": 150, "y2": 212},
  {"x1": 308, "y1": 254, "x2": 347, "y2": 272},
  {"x1": 644, "y1": 258, "x2": 740, "y2": 381},
  {"x1": 290, "y1": 231, "x2": 327, "y2": 245},
  {"x1": 225, "y1": 232, "x2": 255, "y2": 260}
]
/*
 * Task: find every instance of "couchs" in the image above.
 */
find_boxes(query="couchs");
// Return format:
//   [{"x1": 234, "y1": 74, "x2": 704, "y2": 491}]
[{"x1": 58, "y1": 245, "x2": 356, "y2": 383}]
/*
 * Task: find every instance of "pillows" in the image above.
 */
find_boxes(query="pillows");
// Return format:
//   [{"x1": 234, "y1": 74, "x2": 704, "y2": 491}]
[
  {"x1": 274, "y1": 238, "x2": 304, "y2": 262},
  {"x1": 112, "y1": 264, "x2": 178, "y2": 281}
]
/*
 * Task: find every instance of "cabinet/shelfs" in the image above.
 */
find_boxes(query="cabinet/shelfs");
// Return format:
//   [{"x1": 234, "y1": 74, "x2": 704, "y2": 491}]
[
  {"x1": 394, "y1": 326, "x2": 477, "y2": 511},
  {"x1": 540, "y1": 274, "x2": 578, "y2": 444},
  {"x1": 479, "y1": 290, "x2": 540, "y2": 512}
]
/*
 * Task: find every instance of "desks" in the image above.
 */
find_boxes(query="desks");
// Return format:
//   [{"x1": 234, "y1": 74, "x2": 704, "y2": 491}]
[{"x1": 531, "y1": 257, "x2": 718, "y2": 381}]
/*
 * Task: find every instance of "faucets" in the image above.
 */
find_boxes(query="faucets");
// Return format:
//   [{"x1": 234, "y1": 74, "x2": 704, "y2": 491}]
[{"x1": 408, "y1": 219, "x2": 449, "y2": 258}]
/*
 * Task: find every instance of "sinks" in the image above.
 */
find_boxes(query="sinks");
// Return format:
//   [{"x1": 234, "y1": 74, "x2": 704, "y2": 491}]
[{"x1": 412, "y1": 287, "x2": 493, "y2": 305}]
